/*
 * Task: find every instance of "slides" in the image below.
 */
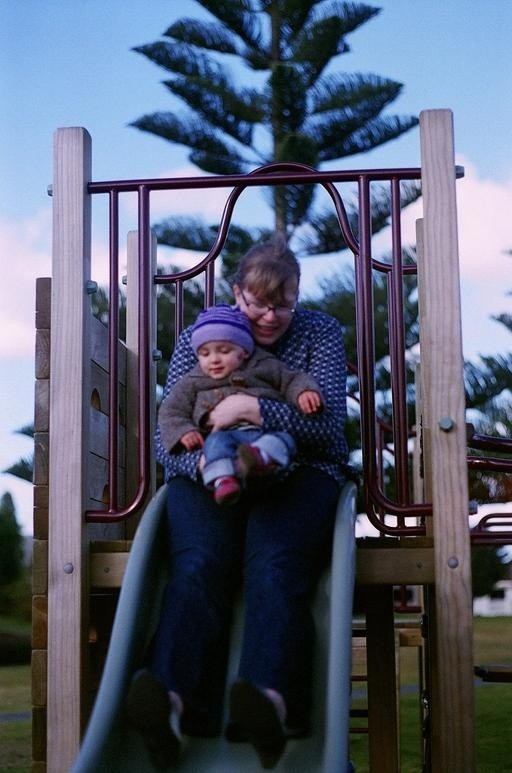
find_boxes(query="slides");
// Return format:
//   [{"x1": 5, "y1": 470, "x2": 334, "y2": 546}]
[{"x1": 69, "y1": 478, "x2": 361, "y2": 770}]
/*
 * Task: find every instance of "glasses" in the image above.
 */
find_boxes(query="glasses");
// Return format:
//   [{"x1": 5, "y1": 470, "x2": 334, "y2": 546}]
[{"x1": 241, "y1": 290, "x2": 298, "y2": 319}]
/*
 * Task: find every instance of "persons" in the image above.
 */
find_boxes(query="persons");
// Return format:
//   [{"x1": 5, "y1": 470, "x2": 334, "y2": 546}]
[
  {"x1": 125, "y1": 233, "x2": 349, "y2": 773},
  {"x1": 157, "y1": 303, "x2": 326, "y2": 505}
]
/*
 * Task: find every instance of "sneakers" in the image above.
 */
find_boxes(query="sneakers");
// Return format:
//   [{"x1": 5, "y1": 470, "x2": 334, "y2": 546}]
[
  {"x1": 126, "y1": 669, "x2": 191, "y2": 770},
  {"x1": 213, "y1": 476, "x2": 241, "y2": 507},
  {"x1": 237, "y1": 444, "x2": 274, "y2": 488},
  {"x1": 227, "y1": 677, "x2": 290, "y2": 768}
]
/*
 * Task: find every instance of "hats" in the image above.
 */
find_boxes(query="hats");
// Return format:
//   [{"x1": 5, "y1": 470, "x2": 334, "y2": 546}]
[{"x1": 187, "y1": 303, "x2": 255, "y2": 359}]
